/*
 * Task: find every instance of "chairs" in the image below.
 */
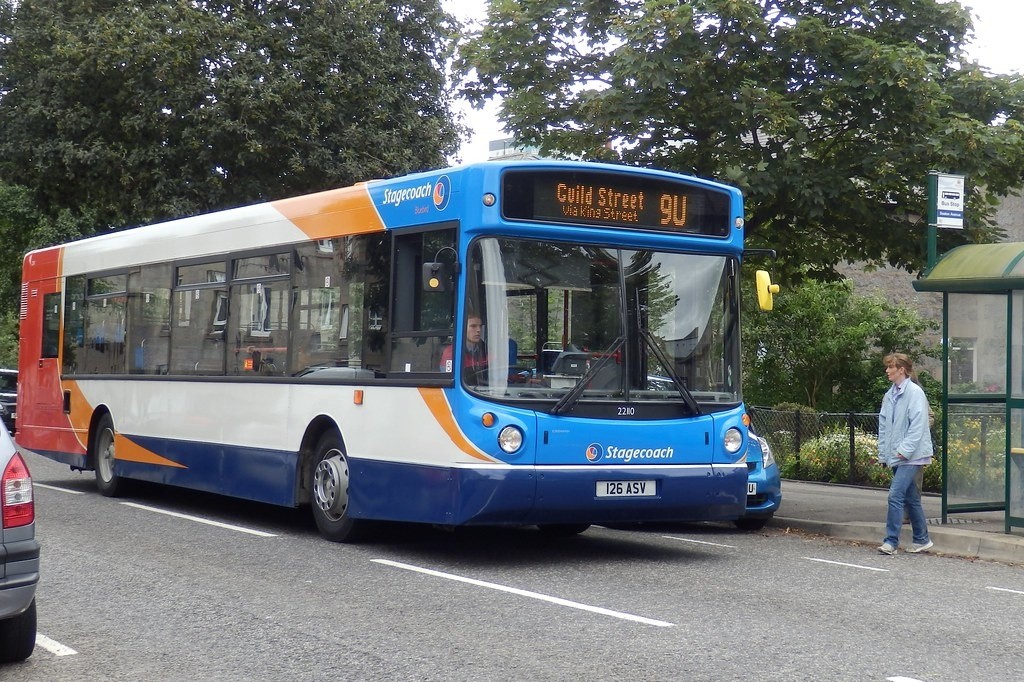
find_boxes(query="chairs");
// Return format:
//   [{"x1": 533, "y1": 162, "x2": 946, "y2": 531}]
[{"x1": 140, "y1": 322, "x2": 321, "y2": 377}]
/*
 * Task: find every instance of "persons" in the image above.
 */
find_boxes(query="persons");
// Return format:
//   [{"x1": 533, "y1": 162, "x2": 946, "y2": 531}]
[
  {"x1": 878, "y1": 355, "x2": 935, "y2": 557},
  {"x1": 439, "y1": 311, "x2": 526, "y2": 383}
]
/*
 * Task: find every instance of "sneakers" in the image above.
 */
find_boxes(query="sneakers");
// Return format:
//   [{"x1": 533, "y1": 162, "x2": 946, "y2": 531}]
[
  {"x1": 878, "y1": 544, "x2": 897, "y2": 556},
  {"x1": 906, "y1": 536, "x2": 933, "y2": 553}
]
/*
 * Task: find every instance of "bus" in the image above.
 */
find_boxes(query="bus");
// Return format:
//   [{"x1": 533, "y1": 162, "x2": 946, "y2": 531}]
[{"x1": 14, "y1": 156, "x2": 779, "y2": 545}]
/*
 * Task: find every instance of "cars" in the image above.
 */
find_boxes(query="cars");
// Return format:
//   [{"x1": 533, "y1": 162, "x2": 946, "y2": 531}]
[
  {"x1": 645, "y1": 374, "x2": 782, "y2": 532},
  {"x1": 0, "y1": 368, "x2": 20, "y2": 434},
  {"x1": 0, "y1": 402, "x2": 41, "y2": 665}
]
